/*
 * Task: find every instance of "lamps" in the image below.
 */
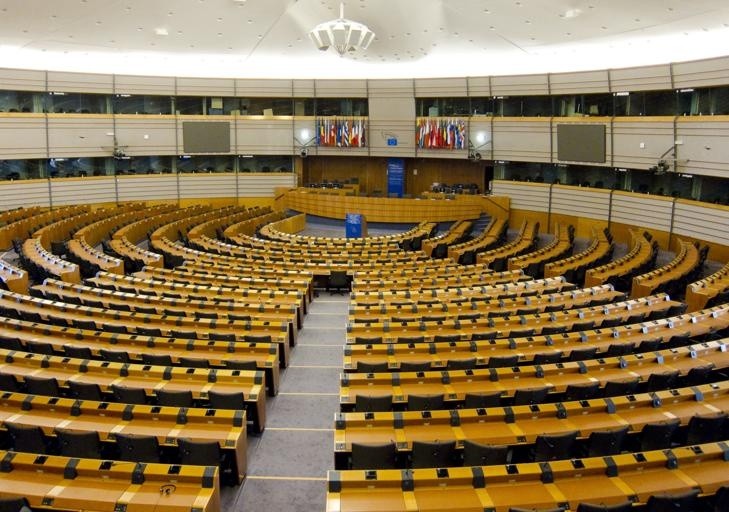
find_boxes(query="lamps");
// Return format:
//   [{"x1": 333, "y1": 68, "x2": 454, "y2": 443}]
[{"x1": 304, "y1": 0, "x2": 373, "y2": 58}]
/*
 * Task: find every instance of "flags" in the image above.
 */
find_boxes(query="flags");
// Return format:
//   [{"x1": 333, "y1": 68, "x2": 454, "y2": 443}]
[
  {"x1": 416, "y1": 117, "x2": 465, "y2": 150},
  {"x1": 315, "y1": 112, "x2": 368, "y2": 148}
]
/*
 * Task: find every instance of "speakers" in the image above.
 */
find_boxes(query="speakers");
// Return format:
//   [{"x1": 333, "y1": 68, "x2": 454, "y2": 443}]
[{"x1": 310, "y1": 22, "x2": 376, "y2": 57}]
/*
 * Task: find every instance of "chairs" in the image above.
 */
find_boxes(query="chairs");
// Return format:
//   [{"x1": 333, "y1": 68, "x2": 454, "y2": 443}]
[
  {"x1": 325, "y1": 311, "x2": 728, "y2": 510},
  {"x1": 0, "y1": 178, "x2": 729, "y2": 315},
  {"x1": 1, "y1": 306, "x2": 299, "y2": 510}
]
